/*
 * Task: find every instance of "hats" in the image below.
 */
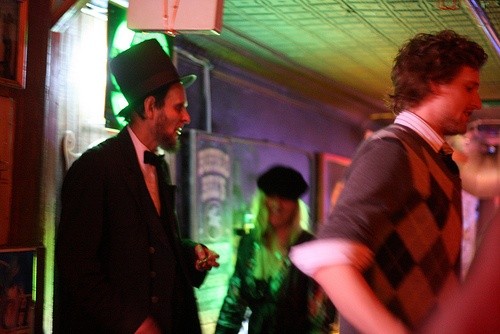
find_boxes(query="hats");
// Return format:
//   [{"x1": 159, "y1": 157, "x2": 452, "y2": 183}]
[
  {"x1": 111, "y1": 36, "x2": 197, "y2": 117},
  {"x1": 257, "y1": 163, "x2": 310, "y2": 200}
]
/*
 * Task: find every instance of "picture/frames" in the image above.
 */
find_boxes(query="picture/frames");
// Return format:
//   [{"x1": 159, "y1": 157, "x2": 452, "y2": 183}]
[{"x1": 317, "y1": 152, "x2": 351, "y2": 225}]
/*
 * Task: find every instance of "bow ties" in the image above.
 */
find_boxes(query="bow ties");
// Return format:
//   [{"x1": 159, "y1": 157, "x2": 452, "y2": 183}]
[{"x1": 143, "y1": 150, "x2": 164, "y2": 167}]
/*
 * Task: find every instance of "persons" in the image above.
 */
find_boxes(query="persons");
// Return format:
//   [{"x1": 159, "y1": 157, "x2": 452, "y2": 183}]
[
  {"x1": 52, "y1": 39, "x2": 220, "y2": 334},
  {"x1": 214, "y1": 31, "x2": 500, "y2": 334}
]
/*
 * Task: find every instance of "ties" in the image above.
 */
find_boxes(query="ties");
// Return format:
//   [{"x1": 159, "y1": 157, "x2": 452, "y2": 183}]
[{"x1": 440, "y1": 149, "x2": 460, "y2": 176}]
